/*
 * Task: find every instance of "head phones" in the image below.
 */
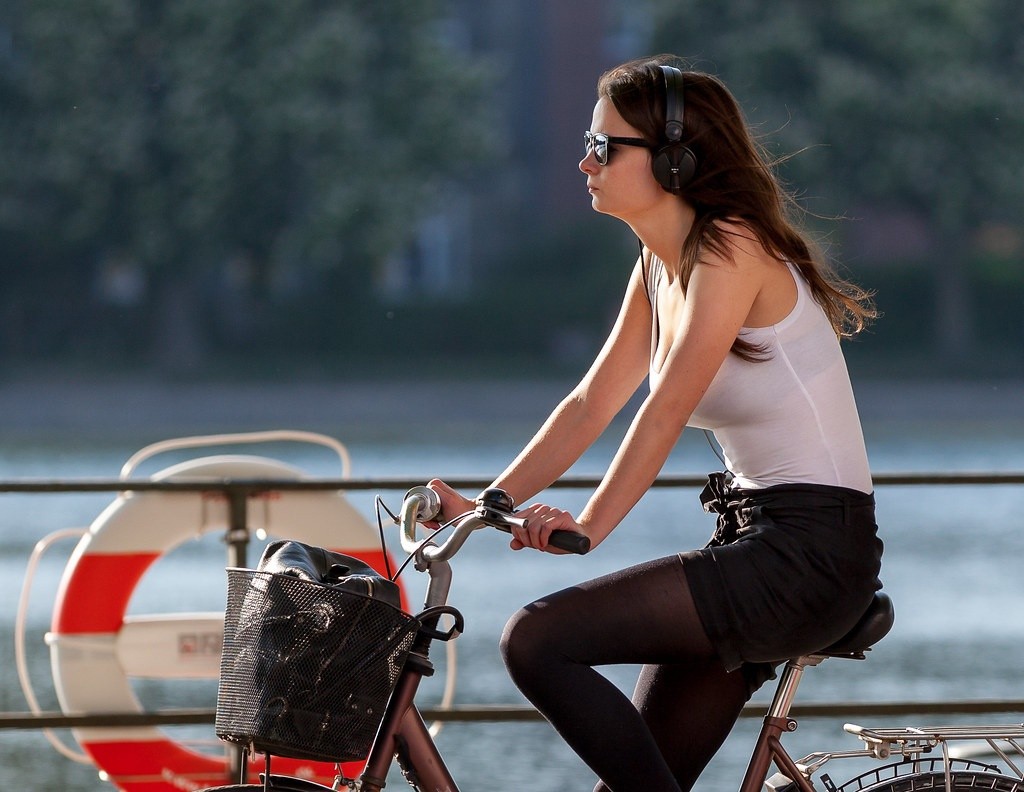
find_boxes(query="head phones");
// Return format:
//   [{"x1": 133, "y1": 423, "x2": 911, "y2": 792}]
[{"x1": 652, "y1": 65, "x2": 696, "y2": 195}]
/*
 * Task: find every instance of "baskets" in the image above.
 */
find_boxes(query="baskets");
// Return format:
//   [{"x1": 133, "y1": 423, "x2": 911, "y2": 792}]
[{"x1": 215, "y1": 568, "x2": 420, "y2": 761}]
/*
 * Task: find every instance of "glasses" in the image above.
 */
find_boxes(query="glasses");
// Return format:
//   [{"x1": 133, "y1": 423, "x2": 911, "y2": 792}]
[{"x1": 584, "y1": 131, "x2": 646, "y2": 165}]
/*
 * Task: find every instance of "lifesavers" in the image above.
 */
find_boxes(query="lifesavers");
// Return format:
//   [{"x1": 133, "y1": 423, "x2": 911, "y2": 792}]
[{"x1": 48, "y1": 455, "x2": 412, "y2": 792}]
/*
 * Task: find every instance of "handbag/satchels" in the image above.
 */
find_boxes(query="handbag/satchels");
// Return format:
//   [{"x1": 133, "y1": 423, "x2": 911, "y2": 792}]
[{"x1": 216, "y1": 539, "x2": 463, "y2": 762}]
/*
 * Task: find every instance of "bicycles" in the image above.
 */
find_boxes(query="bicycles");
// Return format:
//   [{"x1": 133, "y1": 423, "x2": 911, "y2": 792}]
[{"x1": 194, "y1": 484, "x2": 1023, "y2": 792}]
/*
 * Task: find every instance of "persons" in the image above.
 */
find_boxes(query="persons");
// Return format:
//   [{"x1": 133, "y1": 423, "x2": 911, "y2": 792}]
[{"x1": 413, "y1": 56, "x2": 886, "y2": 792}]
